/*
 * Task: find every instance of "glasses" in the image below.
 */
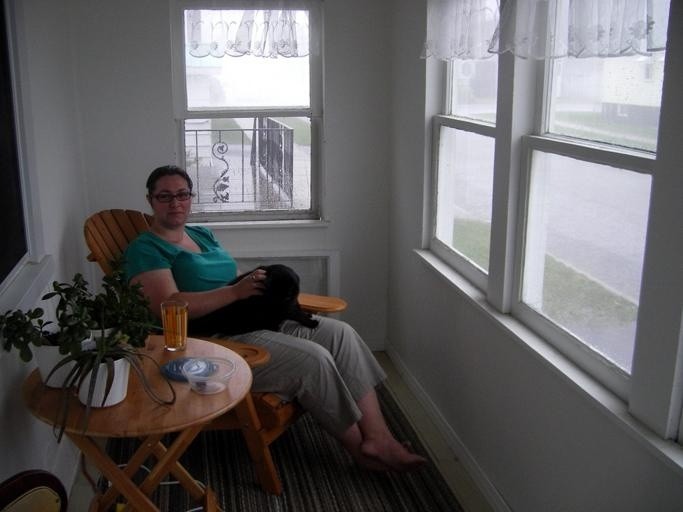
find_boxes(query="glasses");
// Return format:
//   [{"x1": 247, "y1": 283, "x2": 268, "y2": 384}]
[{"x1": 149, "y1": 192, "x2": 196, "y2": 203}]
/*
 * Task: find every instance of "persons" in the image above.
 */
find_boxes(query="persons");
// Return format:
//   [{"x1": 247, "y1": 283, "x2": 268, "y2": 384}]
[{"x1": 123, "y1": 165, "x2": 430, "y2": 480}]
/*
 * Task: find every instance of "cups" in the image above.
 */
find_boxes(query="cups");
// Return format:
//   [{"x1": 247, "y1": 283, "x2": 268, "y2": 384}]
[{"x1": 161, "y1": 299, "x2": 189, "y2": 352}]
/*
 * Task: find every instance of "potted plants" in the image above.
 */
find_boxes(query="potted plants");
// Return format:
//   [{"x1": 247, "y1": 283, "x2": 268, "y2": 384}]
[{"x1": 0, "y1": 247, "x2": 171, "y2": 412}]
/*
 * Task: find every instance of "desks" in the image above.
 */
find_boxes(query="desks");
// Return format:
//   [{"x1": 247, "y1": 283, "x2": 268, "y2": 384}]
[{"x1": 23, "y1": 331, "x2": 254, "y2": 512}]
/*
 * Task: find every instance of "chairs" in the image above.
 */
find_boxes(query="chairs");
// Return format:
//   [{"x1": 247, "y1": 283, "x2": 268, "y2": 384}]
[{"x1": 84, "y1": 208, "x2": 351, "y2": 496}]
